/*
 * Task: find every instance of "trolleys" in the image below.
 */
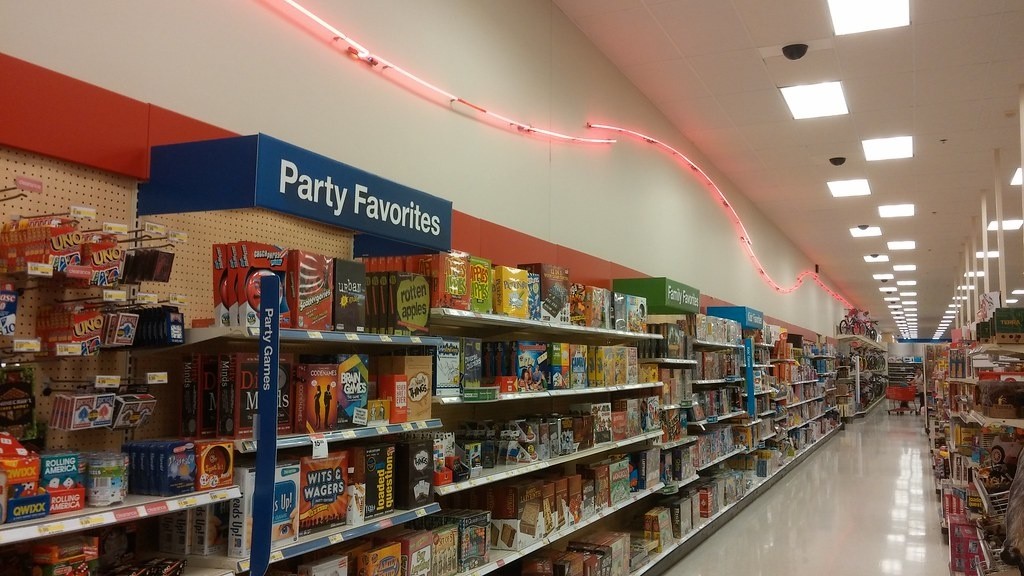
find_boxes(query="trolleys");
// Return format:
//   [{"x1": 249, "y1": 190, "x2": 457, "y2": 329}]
[{"x1": 885, "y1": 382, "x2": 919, "y2": 416}]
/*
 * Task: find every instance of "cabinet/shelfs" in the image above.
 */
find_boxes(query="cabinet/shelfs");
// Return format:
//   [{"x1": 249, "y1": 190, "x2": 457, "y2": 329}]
[
  {"x1": 890, "y1": 308, "x2": 1024, "y2": 576},
  {"x1": 833, "y1": 331, "x2": 890, "y2": 423},
  {"x1": 612, "y1": 276, "x2": 772, "y2": 556},
  {"x1": 706, "y1": 301, "x2": 846, "y2": 486},
  {"x1": 131, "y1": 240, "x2": 442, "y2": 576},
  {"x1": 354, "y1": 253, "x2": 681, "y2": 576}
]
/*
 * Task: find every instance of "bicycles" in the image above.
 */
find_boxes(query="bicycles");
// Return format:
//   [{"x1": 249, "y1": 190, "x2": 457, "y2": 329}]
[
  {"x1": 839, "y1": 308, "x2": 863, "y2": 335},
  {"x1": 858, "y1": 319, "x2": 880, "y2": 342}
]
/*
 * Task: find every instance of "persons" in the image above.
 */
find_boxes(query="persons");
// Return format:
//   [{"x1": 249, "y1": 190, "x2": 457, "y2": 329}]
[{"x1": 908, "y1": 367, "x2": 925, "y2": 416}]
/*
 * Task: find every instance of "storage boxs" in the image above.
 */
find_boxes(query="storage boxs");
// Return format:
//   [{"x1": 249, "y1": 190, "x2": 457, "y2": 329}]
[
  {"x1": 506, "y1": 448, "x2": 780, "y2": 576},
  {"x1": 138, "y1": 241, "x2": 490, "y2": 576},
  {"x1": 570, "y1": 396, "x2": 660, "y2": 450},
  {"x1": 359, "y1": 256, "x2": 647, "y2": 332},
  {"x1": 380, "y1": 340, "x2": 658, "y2": 386}
]
